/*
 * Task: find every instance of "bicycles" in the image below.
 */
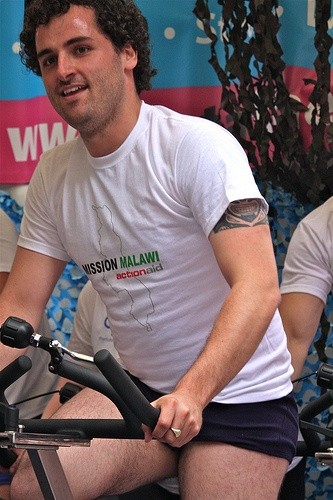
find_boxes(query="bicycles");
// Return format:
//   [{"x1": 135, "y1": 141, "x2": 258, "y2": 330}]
[{"x1": 0, "y1": 316, "x2": 310, "y2": 500}]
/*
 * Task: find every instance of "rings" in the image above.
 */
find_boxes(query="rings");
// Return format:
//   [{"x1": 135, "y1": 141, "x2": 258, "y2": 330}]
[{"x1": 171, "y1": 426, "x2": 183, "y2": 437}]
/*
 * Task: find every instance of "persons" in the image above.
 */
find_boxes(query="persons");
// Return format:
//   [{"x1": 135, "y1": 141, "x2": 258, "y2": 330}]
[
  {"x1": 0, "y1": 205, "x2": 66, "y2": 433},
  {"x1": 11, "y1": 279, "x2": 131, "y2": 478},
  {"x1": 0, "y1": 0, "x2": 297, "y2": 499},
  {"x1": 280, "y1": 192, "x2": 331, "y2": 389}
]
